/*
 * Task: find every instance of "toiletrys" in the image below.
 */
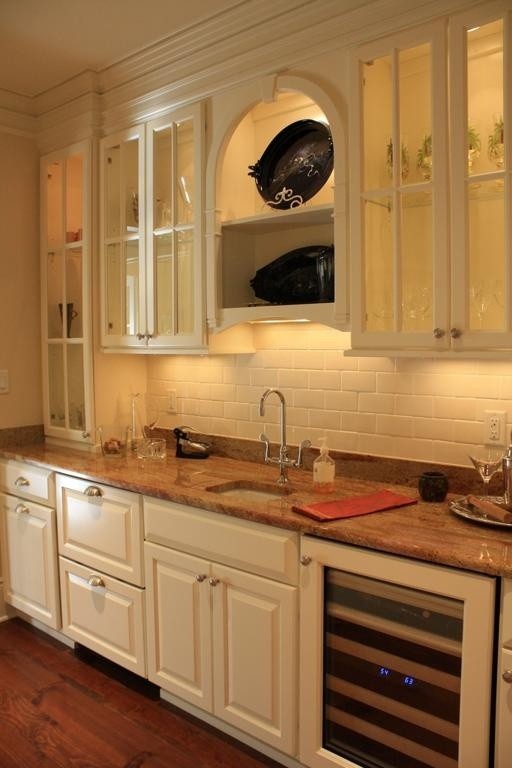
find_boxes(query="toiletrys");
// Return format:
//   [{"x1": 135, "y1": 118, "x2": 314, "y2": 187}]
[{"x1": 313, "y1": 435, "x2": 336, "y2": 485}]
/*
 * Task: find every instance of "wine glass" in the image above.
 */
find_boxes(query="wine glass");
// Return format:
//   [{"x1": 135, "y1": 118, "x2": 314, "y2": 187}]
[
  {"x1": 401, "y1": 286, "x2": 430, "y2": 333},
  {"x1": 468, "y1": 286, "x2": 492, "y2": 327},
  {"x1": 470, "y1": 451, "x2": 503, "y2": 503}
]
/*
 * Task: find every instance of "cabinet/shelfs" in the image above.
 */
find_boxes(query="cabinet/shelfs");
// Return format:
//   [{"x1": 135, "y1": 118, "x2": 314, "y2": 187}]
[
  {"x1": 99, "y1": 99, "x2": 209, "y2": 356},
  {"x1": 54, "y1": 472, "x2": 141, "y2": 588},
  {"x1": 347, "y1": 5, "x2": 512, "y2": 362},
  {"x1": 3, "y1": 456, "x2": 56, "y2": 507},
  {"x1": 52, "y1": 554, "x2": 142, "y2": 682},
  {"x1": 33, "y1": 65, "x2": 148, "y2": 455},
  {"x1": 1, "y1": 493, "x2": 61, "y2": 638},
  {"x1": 296, "y1": 526, "x2": 510, "y2": 768},
  {"x1": 204, "y1": 46, "x2": 347, "y2": 359},
  {"x1": 139, "y1": 493, "x2": 296, "y2": 763}
]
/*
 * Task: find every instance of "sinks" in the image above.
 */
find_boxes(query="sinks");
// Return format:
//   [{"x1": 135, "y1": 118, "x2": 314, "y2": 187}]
[{"x1": 204, "y1": 479, "x2": 298, "y2": 505}]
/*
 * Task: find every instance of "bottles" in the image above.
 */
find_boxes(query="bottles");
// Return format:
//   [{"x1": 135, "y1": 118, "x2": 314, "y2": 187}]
[{"x1": 419, "y1": 472, "x2": 449, "y2": 501}]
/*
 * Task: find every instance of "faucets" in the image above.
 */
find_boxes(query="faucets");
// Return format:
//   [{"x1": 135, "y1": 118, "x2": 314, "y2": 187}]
[{"x1": 259, "y1": 389, "x2": 311, "y2": 487}]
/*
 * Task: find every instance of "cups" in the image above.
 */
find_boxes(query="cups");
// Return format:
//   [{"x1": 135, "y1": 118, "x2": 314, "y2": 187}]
[{"x1": 138, "y1": 438, "x2": 166, "y2": 465}]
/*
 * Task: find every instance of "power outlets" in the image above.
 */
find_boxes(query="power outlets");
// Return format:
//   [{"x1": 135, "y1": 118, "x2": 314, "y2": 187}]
[
  {"x1": 482, "y1": 411, "x2": 507, "y2": 449},
  {"x1": 162, "y1": 389, "x2": 178, "y2": 413}
]
